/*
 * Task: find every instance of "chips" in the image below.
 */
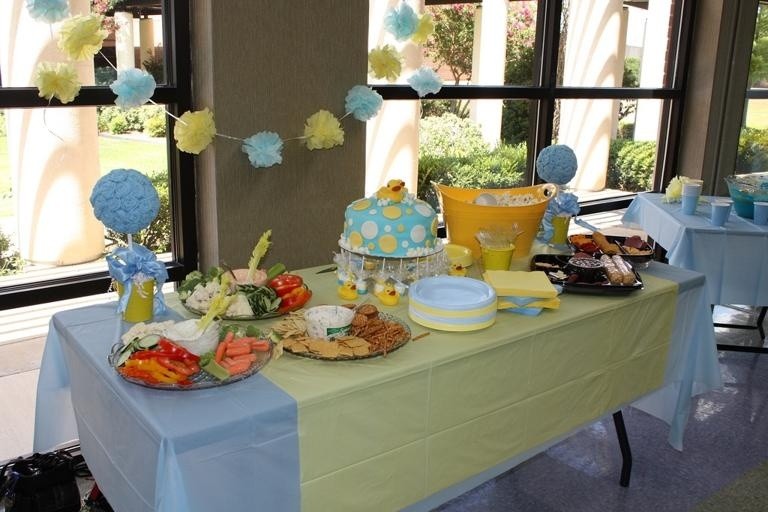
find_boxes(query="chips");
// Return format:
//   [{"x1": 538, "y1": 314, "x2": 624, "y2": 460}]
[{"x1": 273, "y1": 309, "x2": 371, "y2": 359}]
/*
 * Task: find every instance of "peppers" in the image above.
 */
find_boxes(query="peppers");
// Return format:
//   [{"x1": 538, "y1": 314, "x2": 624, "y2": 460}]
[{"x1": 119, "y1": 339, "x2": 201, "y2": 388}]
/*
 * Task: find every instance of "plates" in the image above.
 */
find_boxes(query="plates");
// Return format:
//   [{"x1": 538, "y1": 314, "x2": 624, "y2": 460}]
[
  {"x1": 406, "y1": 276, "x2": 497, "y2": 333},
  {"x1": 180, "y1": 283, "x2": 312, "y2": 322},
  {"x1": 108, "y1": 322, "x2": 272, "y2": 390},
  {"x1": 260, "y1": 312, "x2": 411, "y2": 364}
]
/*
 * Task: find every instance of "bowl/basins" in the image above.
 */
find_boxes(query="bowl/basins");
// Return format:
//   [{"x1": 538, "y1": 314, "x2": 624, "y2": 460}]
[{"x1": 568, "y1": 256, "x2": 602, "y2": 273}]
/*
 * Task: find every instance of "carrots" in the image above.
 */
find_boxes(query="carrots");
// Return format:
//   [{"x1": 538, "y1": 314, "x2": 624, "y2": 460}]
[{"x1": 214, "y1": 329, "x2": 270, "y2": 377}]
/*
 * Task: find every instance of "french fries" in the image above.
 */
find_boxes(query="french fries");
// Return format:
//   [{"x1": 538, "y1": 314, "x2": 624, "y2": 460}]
[{"x1": 350, "y1": 319, "x2": 429, "y2": 357}]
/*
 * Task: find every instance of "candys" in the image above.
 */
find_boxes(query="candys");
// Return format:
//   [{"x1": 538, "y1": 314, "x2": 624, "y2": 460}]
[{"x1": 338, "y1": 252, "x2": 406, "y2": 295}]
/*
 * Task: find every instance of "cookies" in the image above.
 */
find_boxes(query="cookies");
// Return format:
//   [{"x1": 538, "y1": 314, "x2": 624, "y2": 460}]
[{"x1": 342, "y1": 304, "x2": 378, "y2": 328}]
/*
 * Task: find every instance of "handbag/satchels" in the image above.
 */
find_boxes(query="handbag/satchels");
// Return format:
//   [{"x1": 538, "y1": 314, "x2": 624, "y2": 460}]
[{"x1": 6, "y1": 454, "x2": 80, "y2": 512}]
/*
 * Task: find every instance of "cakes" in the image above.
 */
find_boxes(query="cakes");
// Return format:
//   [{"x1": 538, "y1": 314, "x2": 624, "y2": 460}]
[
  {"x1": 396, "y1": 178, "x2": 397, "y2": 179},
  {"x1": 337, "y1": 178, "x2": 444, "y2": 259}
]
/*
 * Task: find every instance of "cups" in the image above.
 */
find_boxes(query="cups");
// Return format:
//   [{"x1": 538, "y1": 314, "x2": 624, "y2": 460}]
[
  {"x1": 709, "y1": 202, "x2": 729, "y2": 226},
  {"x1": 305, "y1": 304, "x2": 353, "y2": 340},
  {"x1": 713, "y1": 198, "x2": 733, "y2": 222},
  {"x1": 481, "y1": 241, "x2": 515, "y2": 270},
  {"x1": 753, "y1": 201, "x2": 768, "y2": 226},
  {"x1": 680, "y1": 179, "x2": 704, "y2": 216},
  {"x1": 551, "y1": 215, "x2": 569, "y2": 245}
]
